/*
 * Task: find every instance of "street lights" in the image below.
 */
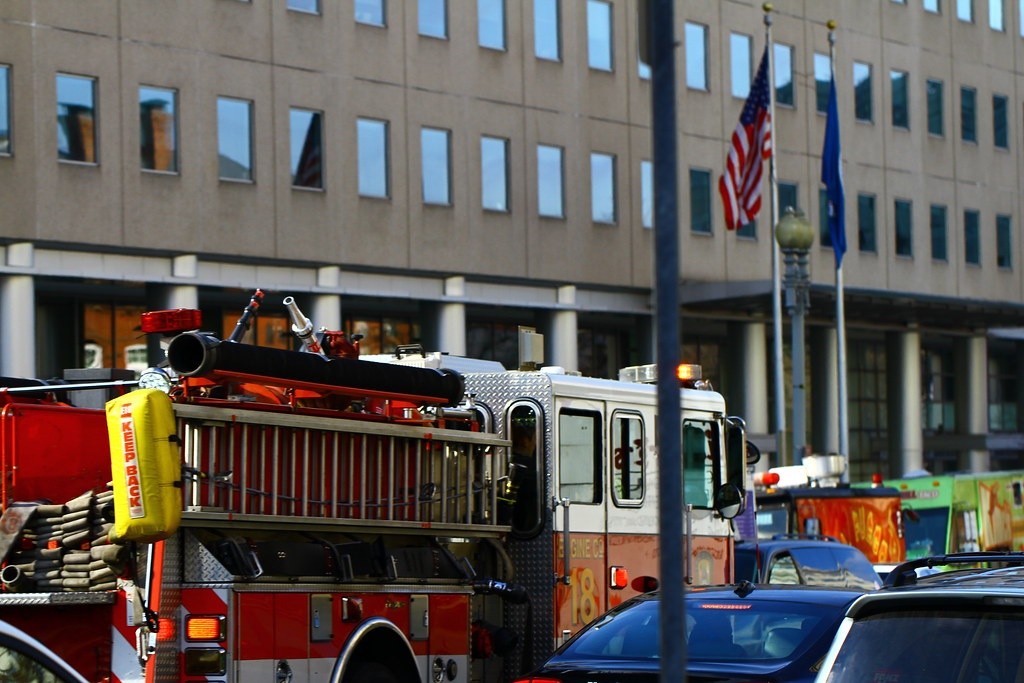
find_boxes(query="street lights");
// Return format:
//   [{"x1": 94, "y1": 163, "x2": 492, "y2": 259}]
[{"x1": 774, "y1": 206, "x2": 816, "y2": 463}]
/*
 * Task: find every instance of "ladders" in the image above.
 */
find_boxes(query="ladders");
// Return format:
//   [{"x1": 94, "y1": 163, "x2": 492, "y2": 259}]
[{"x1": 170, "y1": 400, "x2": 513, "y2": 540}]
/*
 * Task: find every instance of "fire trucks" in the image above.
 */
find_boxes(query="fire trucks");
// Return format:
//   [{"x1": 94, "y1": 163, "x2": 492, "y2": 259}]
[{"x1": 0, "y1": 288, "x2": 763, "y2": 683}]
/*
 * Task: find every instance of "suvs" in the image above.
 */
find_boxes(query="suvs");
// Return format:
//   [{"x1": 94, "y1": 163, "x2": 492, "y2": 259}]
[{"x1": 734, "y1": 533, "x2": 883, "y2": 593}]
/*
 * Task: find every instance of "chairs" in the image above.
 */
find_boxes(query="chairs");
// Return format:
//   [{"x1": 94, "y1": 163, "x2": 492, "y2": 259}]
[
  {"x1": 686, "y1": 644, "x2": 748, "y2": 657},
  {"x1": 762, "y1": 627, "x2": 804, "y2": 656}
]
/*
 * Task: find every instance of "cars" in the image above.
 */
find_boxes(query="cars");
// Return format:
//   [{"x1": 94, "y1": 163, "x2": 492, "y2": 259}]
[
  {"x1": 514, "y1": 581, "x2": 873, "y2": 682},
  {"x1": 811, "y1": 550, "x2": 1024, "y2": 683}
]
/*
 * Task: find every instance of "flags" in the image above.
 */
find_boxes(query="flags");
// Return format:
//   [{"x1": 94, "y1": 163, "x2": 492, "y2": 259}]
[
  {"x1": 717, "y1": 39, "x2": 773, "y2": 231},
  {"x1": 819, "y1": 51, "x2": 848, "y2": 275}
]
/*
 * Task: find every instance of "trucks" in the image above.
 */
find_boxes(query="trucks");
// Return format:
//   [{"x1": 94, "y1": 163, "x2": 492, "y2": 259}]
[
  {"x1": 850, "y1": 470, "x2": 1023, "y2": 585},
  {"x1": 751, "y1": 472, "x2": 905, "y2": 585}
]
